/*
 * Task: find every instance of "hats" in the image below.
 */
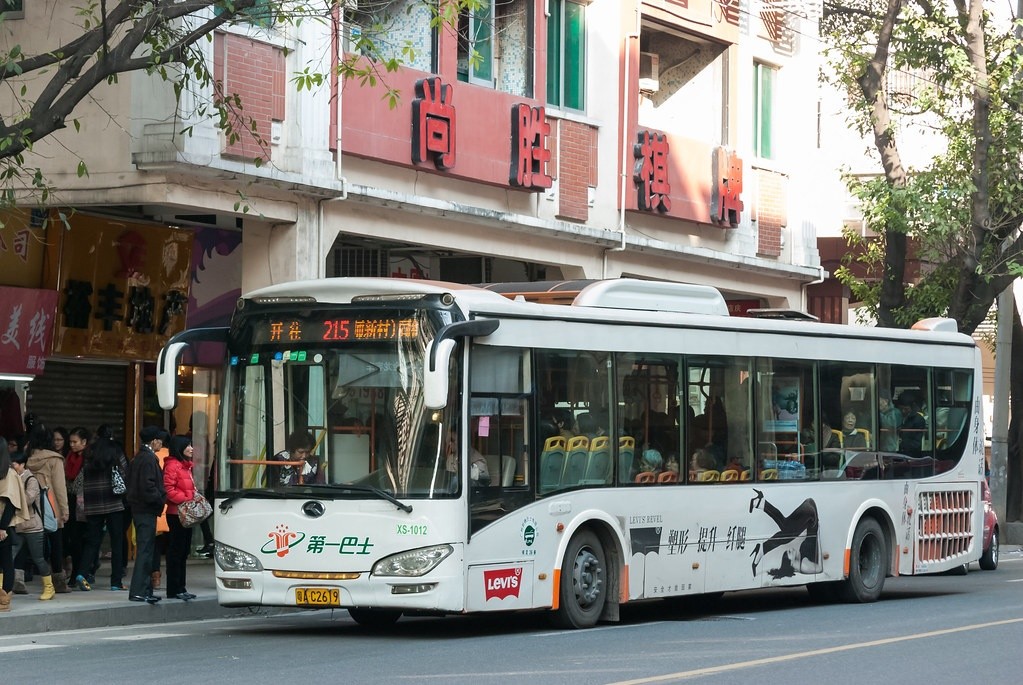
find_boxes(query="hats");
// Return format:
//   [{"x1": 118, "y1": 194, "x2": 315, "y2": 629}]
[
  {"x1": 641, "y1": 449, "x2": 663, "y2": 470},
  {"x1": 24, "y1": 412, "x2": 50, "y2": 435},
  {"x1": 138, "y1": 425, "x2": 167, "y2": 440}
]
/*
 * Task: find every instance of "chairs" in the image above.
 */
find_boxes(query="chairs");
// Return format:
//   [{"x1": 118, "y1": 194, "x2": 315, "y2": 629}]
[
  {"x1": 538, "y1": 403, "x2": 964, "y2": 496},
  {"x1": 483, "y1": 455, "x2": 516, "y2": 487}
]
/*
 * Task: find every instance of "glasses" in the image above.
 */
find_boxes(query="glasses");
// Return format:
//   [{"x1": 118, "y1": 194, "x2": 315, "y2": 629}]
[
  {"x1": 448, "y1": 438, "x2": 457, "y2": 443},
  {"x1": 7, "y1": 442, "x2": 18, "y2": 447}
]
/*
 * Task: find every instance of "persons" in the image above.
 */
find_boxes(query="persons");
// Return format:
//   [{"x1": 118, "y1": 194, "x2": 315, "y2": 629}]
[
  {"x1": 541, "y1": 382, "x2": 952, "y2": 482},
  {"x1": 749, "y1": 488, "x2": 823, "y2": 581},
  {"x1": 266, "y1": 430, "x2": 317, "y2": 488},
  {"x1": 0, "y1": 411, "x2": 212, "y2": 612},
  {"x1": 443, "y1": 423, "x2": 493, "y2": 498}
]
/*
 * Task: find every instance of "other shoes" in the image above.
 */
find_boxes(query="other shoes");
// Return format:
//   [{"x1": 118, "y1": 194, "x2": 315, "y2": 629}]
[
  {"x1": 111, "y1": 577, "x2": 130, "y2": 591},
  {"x1": 167, "y1": 592, "x2": 196, "y2": 601},
  {"x1": 67, "y1": 573, "x2": 97, "y2": 591},
  {"x1": 196, "y1": 544, "x2": 215, "y2": 557}
]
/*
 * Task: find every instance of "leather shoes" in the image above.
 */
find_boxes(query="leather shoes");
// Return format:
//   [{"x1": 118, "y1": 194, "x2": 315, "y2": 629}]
[{"x1": 128, "y1": 594, "x2": 162, "y2": 603}]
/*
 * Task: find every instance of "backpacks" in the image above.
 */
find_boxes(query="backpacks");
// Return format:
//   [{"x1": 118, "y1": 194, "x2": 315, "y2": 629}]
[{"x1": 24, "y1": 476, "x2": 58, "y2": 532}]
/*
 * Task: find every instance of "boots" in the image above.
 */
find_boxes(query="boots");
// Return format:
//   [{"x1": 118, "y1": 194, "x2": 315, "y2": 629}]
[
  {"x1": 39, "y1": 575, "x2": 56, "y2": 600},
  {"x1": 0, "y1": 588, "x2": 12, "y2": 613},
  {"x1": 152, "y1": 571, "x2": 161, "y2": 589},
  {"x1": 51, "y1": 570, "x2": 73, "y2": 593},
  {"x1": 13, "y1": 568, "x2": 31, "y2": 595}
]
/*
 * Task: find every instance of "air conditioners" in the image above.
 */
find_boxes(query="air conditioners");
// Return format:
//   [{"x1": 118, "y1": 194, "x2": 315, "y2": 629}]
[
  {"x1": 640, "y1": 52, "x2": 659, "y2": 94},
  {"x1": 343, "y1": 22, "x2": 362, "y2": 55},
  {"x1": 329, "y1": 247, "x2": 392, "y2": 276}
]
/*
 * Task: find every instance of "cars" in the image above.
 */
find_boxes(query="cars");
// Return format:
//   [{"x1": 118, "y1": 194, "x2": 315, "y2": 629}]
[{"x1": 945, "y1": 478, "x2": 1000, "y2": 575}]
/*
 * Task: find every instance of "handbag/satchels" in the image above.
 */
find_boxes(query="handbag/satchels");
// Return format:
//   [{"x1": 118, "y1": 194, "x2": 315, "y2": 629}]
[
  {"x1": 177, "y1": 489, "x2": 213, "y2": 529},
  {"x1": 110, "y1": 465, "x2": 126, "y2": 495}
]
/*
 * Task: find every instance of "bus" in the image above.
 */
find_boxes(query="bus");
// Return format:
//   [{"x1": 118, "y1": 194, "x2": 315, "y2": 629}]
[{"x1": 157, "y1": 277, "x2": 986, "y2": 631}]
